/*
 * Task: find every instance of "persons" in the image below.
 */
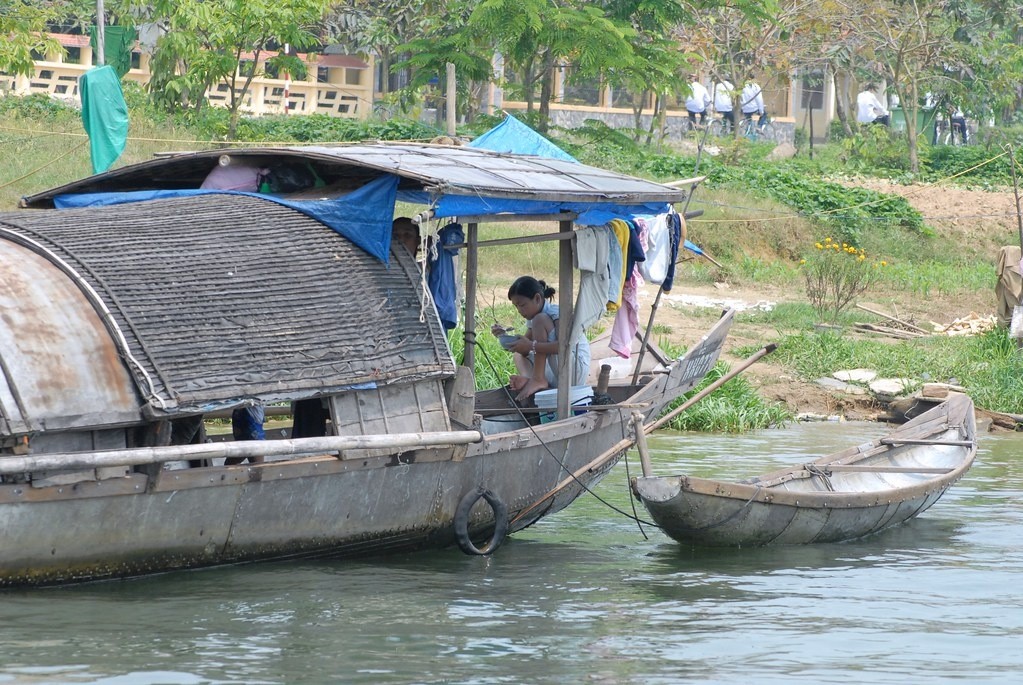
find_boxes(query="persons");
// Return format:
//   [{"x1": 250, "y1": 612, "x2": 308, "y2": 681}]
[
  {"x1": 393, "y1": 217, "x2": 421, "y2": 259},
  {"x1": 684, "y1": 76, "x2": 711, "y2": 130},
  {"x1": 224, "y1": 404, "x2": 265, "y2": 465},
  {"x1": 491, "y1": 276, "x2": 591, "y2": 401},
  {"x1": 926, "y1": 91, "x2": 973, "y2": 146},
  {"x1": 739, "y1": 74, "x2": 767, "y2": 135},
  {"x1": 714, "y1": 74, "x2": 736, "y2": 131},
  {"x1": 857, "y1": 83, "x2": 889, "y2": 126}
]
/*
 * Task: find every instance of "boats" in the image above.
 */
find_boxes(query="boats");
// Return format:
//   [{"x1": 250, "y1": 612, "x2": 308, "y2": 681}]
[
  {"x1": 1, "y1": 140, "x2": 738, "y2": 588},
  {"x1": 630, "y1": 381, "x2": 981, "y2": 551}
]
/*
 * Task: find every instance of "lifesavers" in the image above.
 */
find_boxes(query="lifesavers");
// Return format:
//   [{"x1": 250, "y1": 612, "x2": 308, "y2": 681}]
[{"x1": 449, "y1": 486, "x2": 502, "y2": 557}]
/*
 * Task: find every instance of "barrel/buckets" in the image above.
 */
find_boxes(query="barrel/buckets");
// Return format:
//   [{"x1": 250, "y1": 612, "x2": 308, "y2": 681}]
[{"x1": 534, "y1": 385, "x2": 594, "y2": 425}]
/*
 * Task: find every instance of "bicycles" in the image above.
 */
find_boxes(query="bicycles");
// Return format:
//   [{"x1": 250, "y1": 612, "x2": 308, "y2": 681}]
[
  {"x1": 737, "y1": 110, "x2": 777, "y2": 143},
  {"x1": 680, "y1": 114, "x2": 735, "y2": 142},
  {"x1": 860, "y1": 113, "x2": 890, "y2": 133},
  {"x1": 936, "y1": 120, "x2": 980, "y2": 146}
]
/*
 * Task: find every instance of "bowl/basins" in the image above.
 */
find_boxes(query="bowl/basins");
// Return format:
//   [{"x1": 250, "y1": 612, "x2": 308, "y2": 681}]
[{"x1": 498, "y1": 336, "x2": 519, "y2": 350}]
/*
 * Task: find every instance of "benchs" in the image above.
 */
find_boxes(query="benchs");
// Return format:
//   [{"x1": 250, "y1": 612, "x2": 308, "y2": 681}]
[
  {"x1": 881, "y1": 438, "x2": 972, "y2": 446},
  {"x1": 806, "y1": 463, "x2": 955, "y2": 474},
  {"x1": 476, "y1": 402, "x2": 650, "y2": 409}
]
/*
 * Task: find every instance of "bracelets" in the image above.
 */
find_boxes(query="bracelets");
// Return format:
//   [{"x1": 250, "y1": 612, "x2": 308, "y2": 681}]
[{"x1": 533, "y1": 340, "x2": 537, "y2": 355}]
